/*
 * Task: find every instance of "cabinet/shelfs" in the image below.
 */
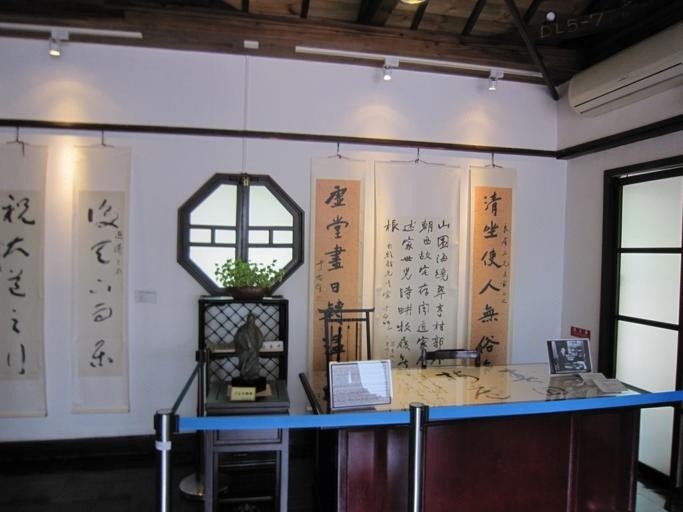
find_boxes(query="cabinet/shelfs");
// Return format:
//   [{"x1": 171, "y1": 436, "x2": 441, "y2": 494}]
[{"x1": 197, "y1": 294, "x2": 289, "y2": 473}]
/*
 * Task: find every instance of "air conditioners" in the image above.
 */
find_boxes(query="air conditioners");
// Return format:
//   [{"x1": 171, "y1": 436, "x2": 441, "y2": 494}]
[{"x1": 568, "y1": 22, "x2": 683, "y2": 120}]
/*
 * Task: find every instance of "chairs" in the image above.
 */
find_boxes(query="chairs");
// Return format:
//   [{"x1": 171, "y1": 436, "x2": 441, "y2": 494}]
[{"x1": 421, "y1": 346, "x2": 482, "y2": 369}]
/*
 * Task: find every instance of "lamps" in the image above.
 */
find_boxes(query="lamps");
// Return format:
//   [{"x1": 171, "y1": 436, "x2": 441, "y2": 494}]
[
  {"x1": 379, "y1": 58, "x2": 505, "y2": 91},
  {"x1": 45, "y1": 29, "x2": 68, "y2": 55}
]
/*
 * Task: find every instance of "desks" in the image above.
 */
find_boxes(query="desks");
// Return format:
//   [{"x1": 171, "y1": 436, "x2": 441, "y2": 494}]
[
  {"x1": 298, "y1": 361, "x2": 649, "y2": 512},
  {"x1": 202, "y1": 380, "x2": 291, "y2": 512}
]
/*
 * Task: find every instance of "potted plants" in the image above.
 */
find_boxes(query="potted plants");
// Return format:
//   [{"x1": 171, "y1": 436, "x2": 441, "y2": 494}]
[{"x1": 213, "y1": 256, "x2": 286, "y2": 300}]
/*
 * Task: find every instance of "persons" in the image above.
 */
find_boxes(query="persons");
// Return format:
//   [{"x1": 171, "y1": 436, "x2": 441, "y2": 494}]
[{"x1": 234, "y1": 314, "x2": 263, "y2": 379}]
